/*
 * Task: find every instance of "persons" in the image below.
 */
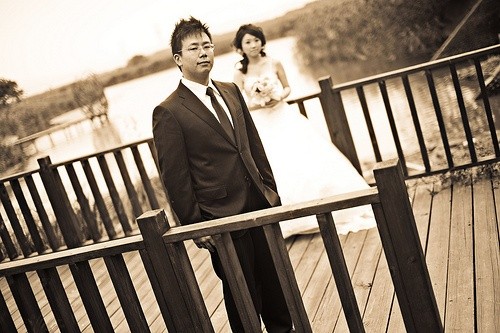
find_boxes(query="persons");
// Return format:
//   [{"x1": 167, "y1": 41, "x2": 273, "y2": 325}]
[
  {"x1": 151, "y1": 16, "x2": 295, "y2": 333},
  {"x1": 231, "y1": 24, "x2": 377, "y2": 241}
]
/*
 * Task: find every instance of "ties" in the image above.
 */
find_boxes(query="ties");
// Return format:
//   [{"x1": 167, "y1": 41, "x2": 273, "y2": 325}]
[{"x1": 206, "y1": 86, "x2": 237, "y2": 148}]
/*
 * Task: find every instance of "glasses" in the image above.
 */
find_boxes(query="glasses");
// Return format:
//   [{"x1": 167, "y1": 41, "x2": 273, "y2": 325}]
[{"x1": 174, "y1": 44, "x2": 215, "y2": 55}]
